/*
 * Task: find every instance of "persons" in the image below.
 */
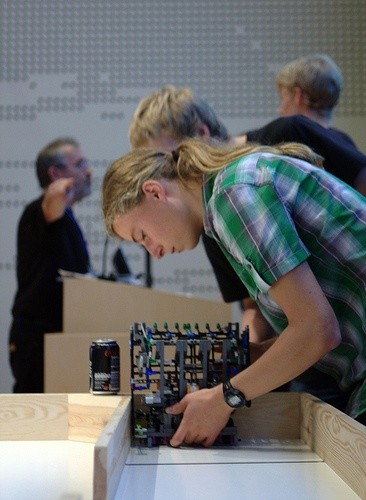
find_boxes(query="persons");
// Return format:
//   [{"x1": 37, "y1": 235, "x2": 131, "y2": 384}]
[
  {"x1": 7, "y1": 138, "x2": 92, "y2": 393},
  {"x1": 129, "y1": 84, "x2": 366, "y2": 360},
  {"x1": 102, "y1": 140, "x2": 366, "y2": 448},
  {"x1": 229, "y1": 54, "x2": 366, "y2": 195}
]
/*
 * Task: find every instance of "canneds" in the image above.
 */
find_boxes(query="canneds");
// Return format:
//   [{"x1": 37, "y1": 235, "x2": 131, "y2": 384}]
[{"x1": 88, "y1": 339, "x2": 120, "y2": 395}]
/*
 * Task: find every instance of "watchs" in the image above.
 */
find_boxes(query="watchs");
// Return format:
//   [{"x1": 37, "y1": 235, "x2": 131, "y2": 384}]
[{"x1": 223, "y1": 377, "x2": 252, "y2": 409}]
